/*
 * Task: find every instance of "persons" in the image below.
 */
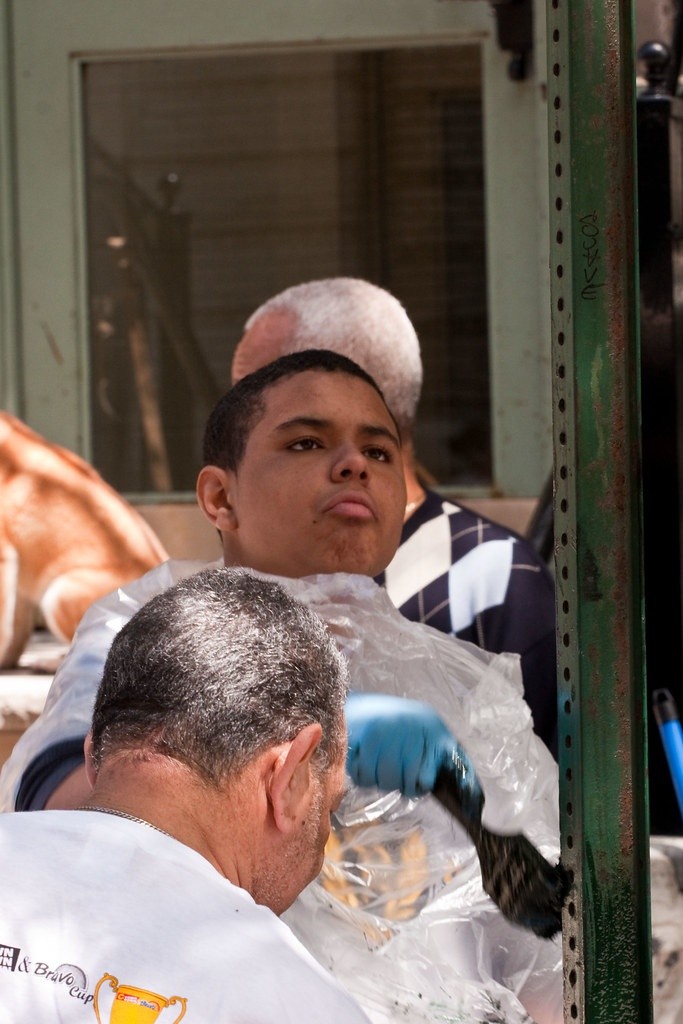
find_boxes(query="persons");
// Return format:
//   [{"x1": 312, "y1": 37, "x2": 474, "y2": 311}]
[{"x1": 3, "y1": 274, "x2": 683, "y2": 1024}]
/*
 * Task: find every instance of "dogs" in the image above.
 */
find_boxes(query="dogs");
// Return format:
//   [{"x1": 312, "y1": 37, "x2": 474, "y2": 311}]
[{"x1": 0, "y1": 409, "x2": 172, "y2": 670}]
